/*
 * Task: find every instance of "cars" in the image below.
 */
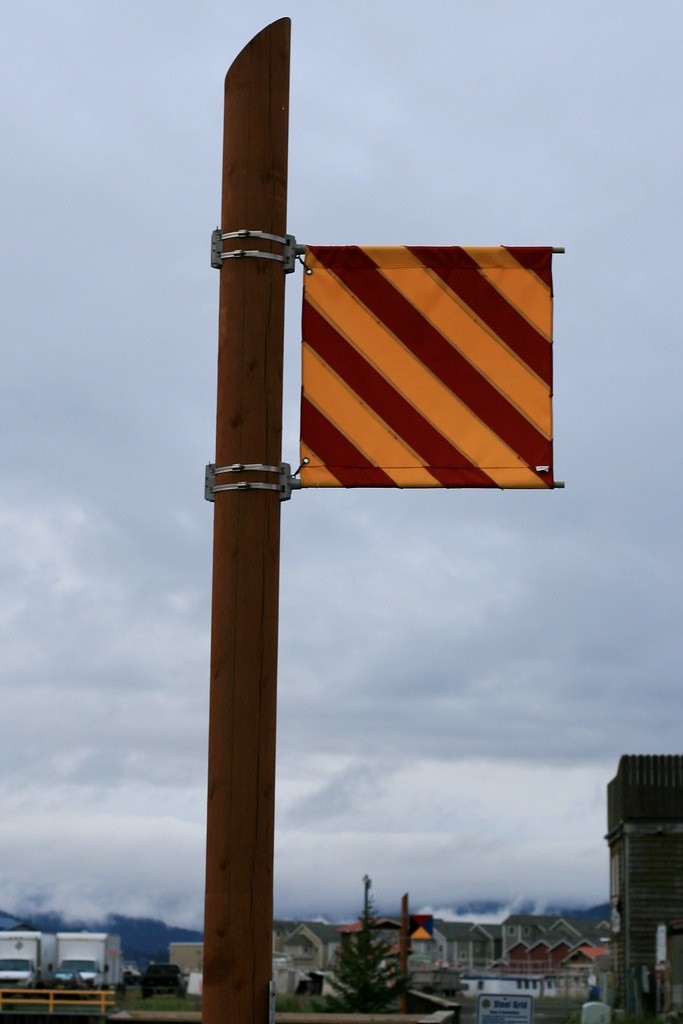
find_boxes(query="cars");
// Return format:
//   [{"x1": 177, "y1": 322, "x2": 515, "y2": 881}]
[{"x1": 140, "y1": 965, "x2": 189, "y2": 999}]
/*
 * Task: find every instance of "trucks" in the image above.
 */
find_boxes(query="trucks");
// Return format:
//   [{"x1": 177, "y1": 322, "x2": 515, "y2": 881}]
[
  {"x1": 56, "y1": 933, "x2": 123, "y2": 992},
  {"x1": 0, "y1": 930, "x2": 57, "y2": 991}
]
